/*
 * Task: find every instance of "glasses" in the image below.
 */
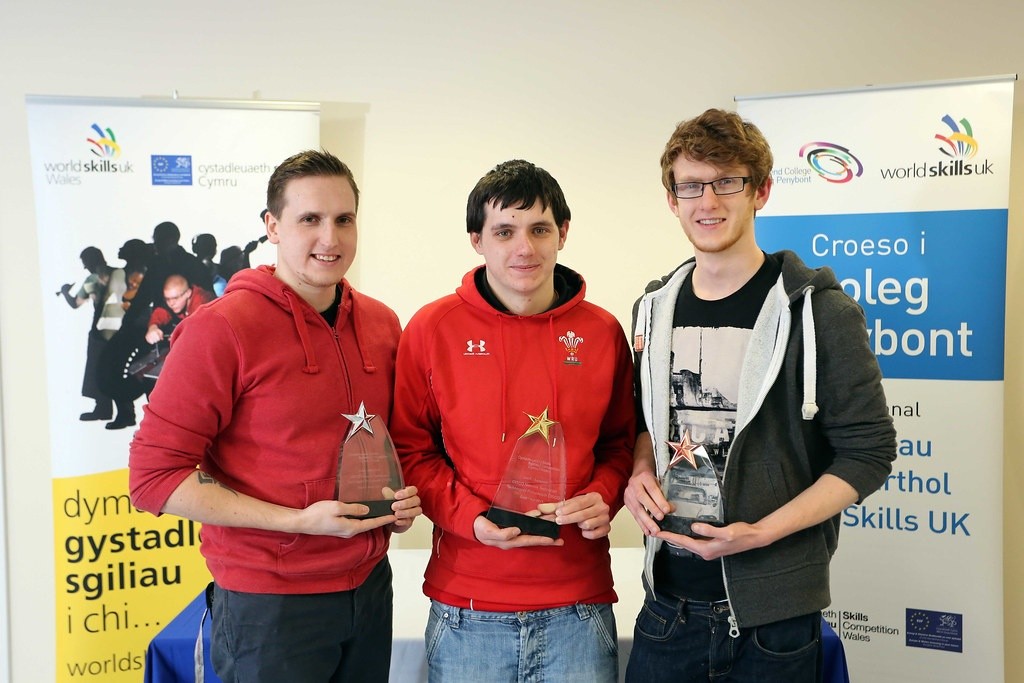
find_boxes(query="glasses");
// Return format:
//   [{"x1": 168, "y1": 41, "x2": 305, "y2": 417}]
[
  {"x1": 162, "y1": 288, "x2": 188, "y2": 302},
  {"x1": 671, "y1": 176, "x2": 752, "y2": 200}
]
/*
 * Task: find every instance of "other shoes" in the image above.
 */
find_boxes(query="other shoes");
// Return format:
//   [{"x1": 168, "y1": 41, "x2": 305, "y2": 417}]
[
  {"x1": 80, "y1": 405, "x2": 113, "y2": 421},
  {"x1": 105, "y1": 413, "x2": 136, "y2": 429}
]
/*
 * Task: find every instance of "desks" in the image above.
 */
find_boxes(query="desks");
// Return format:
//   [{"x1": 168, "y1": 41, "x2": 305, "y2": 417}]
[{"x1": 143, "y1": 546, "x2": 851, "y2": 683}]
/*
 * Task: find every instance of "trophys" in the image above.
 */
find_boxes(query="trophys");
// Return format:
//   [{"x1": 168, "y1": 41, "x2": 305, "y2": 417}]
[
  {"x1": 335, "y1": 400, "x2": 407, "y2": 521},
  {"x1": 648, "y1": 429, "x2": 723, "y2": 542},
  {"x1": 481, "y1": 405, "x2": 568, "y2": 542}
]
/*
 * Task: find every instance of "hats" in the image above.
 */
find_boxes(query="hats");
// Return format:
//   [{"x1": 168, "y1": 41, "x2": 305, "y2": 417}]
[{"x1": 219, "y1": 246, "x2": 245, "y2": 267}]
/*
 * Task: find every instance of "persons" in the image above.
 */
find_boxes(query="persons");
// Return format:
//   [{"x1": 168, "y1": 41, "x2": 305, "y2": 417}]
[
  {"x1": 129, "y1": 149, "x2": 422, "y2": 683},
  {"x1": 392, "y1": 160, "x2": 637, "y2": 683},
  {"x1": 55, "y1": 222, "x2": 246, "y2": 430},
  {"x1": 625, "y1": 107, "x2": 896, "y2": 682}
]
